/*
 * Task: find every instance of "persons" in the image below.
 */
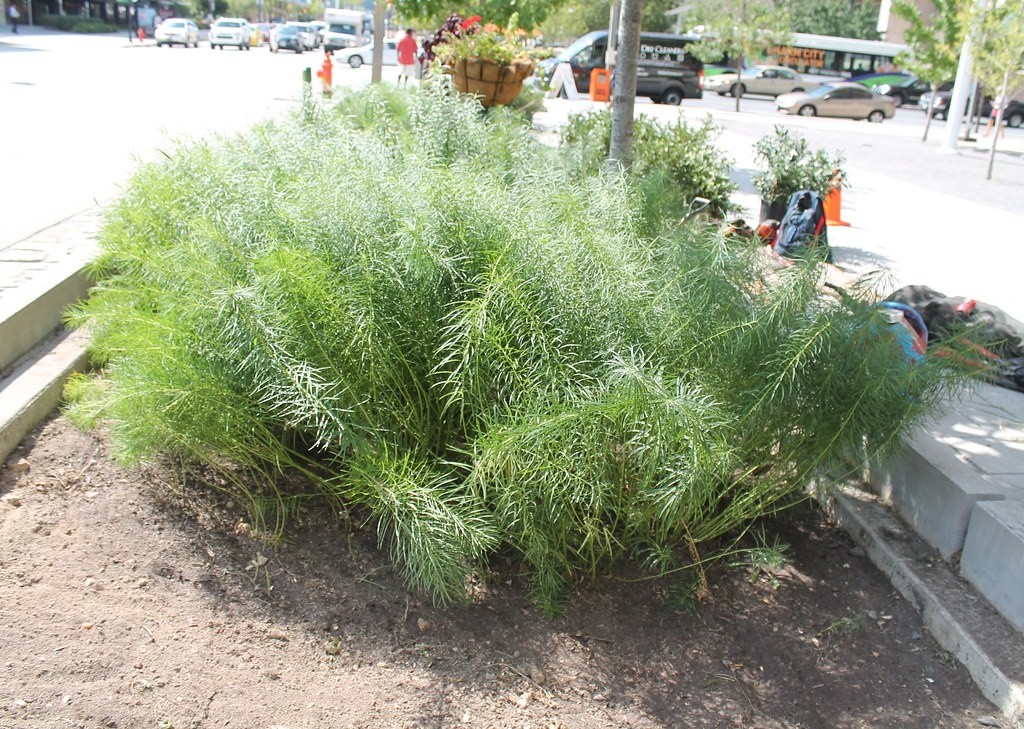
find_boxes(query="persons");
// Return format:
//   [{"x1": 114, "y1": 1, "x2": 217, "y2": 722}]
[
  {"x1": 153, "y1": 14, "x2": 161, "y2": 29},
  {"x1": 983, "y1": 88, "x2": 1008, "y2": 138},
  {"x1": 268, "y1": 25, "x2": 279, "y2": 53},
  {"x1": 395, "y1": 29, "x2": 446, "y2": 90},
  {"x1": 240, "y1": 23, "x2": 249, "y2": 50},
  {"x1": 10, "y1": 4, "x2": 19, "y2": 34}
]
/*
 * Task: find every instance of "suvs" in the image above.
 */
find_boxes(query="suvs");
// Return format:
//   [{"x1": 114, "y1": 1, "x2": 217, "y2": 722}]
[{"x1": 208, "y1": 16, "x2": 251, "y2": 50}]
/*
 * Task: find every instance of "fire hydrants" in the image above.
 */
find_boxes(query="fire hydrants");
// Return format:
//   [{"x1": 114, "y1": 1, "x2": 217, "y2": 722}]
[
  {"x1": 136, "y1": 28, "x2": 147, "y2": 42},
  {"x1": 317, "y1": 52, "x2": 336, "y2": 98}
]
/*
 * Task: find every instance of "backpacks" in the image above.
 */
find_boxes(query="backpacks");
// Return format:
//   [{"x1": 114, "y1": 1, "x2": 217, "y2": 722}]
[
  {"x1": 773, "y1": 190, "x2": 831, "y2": 263},
  {"x1": 881, "y1": 284, "x2": 1024, "y2": 395}
]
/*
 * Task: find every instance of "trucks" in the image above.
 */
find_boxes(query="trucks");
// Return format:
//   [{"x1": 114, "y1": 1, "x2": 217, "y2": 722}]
[{"x1": 324, "y1": 8, "x2": 375, "y2": 56}]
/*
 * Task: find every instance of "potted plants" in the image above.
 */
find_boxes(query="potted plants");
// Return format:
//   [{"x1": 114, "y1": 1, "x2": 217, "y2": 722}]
[
  {"x1": 749, "y1": 122, "x2": 852, "y2": 226},
  {"x1": 432, "y1": 11, "x2": 558, "y2": 110}
]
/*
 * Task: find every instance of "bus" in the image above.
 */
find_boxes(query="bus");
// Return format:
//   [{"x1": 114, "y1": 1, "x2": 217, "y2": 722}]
[{"x1": 685, "y1": 25, "x2": 917, "y2": 78}]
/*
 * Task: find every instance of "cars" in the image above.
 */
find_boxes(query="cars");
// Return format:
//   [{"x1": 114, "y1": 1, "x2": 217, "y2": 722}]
[
  {"x1": 269, "y1": 25, "x2": 304, "y2": 54},
  {"x1": 249, "y1": 17, "x2": 325, "y2": 51},
  {"x1": 699, "y1": 63, "x2": 829, "y2": 100},
  {"x1": 155, "y1": 18, "x2": 200, "y2": 49},
  {"x1": 919, "y1": 87, "x2": 1024, "y2": 128},
  {"x1": 874, "y1": 77, "x2": 957, "y2": 109},
  {"x1": 330, "y1": 39, "x2": 426, "y2": 68},
  {"x1": 774, "y1": 80, "x2": 897, "y2": 125}
]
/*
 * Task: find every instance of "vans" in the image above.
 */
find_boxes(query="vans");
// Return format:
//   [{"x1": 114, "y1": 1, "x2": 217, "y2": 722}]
[{"x1": 533, "y1": 29, "x2": 704, "y2": 106}]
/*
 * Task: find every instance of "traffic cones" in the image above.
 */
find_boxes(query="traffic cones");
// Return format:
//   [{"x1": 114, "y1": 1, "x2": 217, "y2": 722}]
[{"x1": 823, "y1": 174, "x2": 852, "y2": 228}]
